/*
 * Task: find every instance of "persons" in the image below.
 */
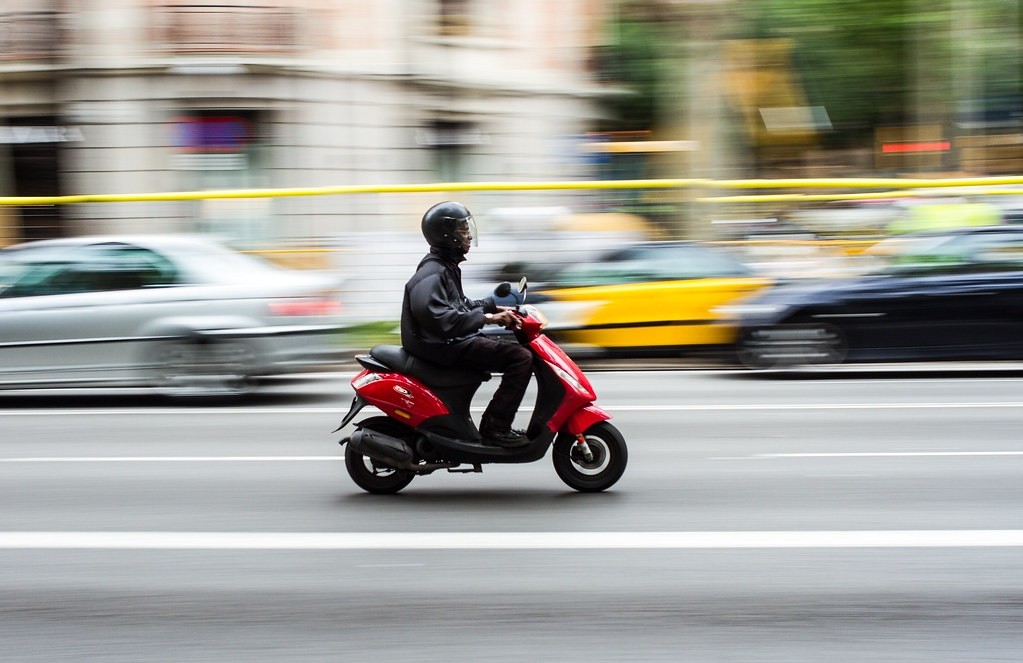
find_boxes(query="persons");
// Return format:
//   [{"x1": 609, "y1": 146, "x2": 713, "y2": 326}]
[{"x1": 398, "y1": 200, "x2": 529, "y2": 447}]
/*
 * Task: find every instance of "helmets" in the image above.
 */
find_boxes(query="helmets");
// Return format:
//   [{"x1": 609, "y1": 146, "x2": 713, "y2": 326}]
[{"x1": 422, "y1": 201, "x2": 471, "y2": 232}]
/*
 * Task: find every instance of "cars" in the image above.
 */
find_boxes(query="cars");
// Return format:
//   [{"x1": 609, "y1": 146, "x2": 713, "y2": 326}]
[
  {"x1": 0, "y1": 234, "x2": 356, "y2": 403},
  {"x1": 517, "y1": 238, "x2": 785, "y2": 353},
  {"x1": 729, "y1": 200, "x2": 1022, "y2": 373}
]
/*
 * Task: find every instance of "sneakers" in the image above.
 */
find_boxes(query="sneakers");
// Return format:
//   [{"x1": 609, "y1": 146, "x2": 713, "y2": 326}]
[{"x1": 483, "y1": 425, "x2": 530, "y2": 450}]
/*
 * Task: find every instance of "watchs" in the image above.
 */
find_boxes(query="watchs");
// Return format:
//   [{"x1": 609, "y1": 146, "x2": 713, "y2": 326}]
[{"x1": 483, "y1": 313, "x2": 494, "y2": 325}]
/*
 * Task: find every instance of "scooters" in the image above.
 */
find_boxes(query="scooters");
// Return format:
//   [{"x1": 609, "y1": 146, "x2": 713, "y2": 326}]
[{"x1": 328, "y1": 275, "x2": 630, "y2": 498}]
